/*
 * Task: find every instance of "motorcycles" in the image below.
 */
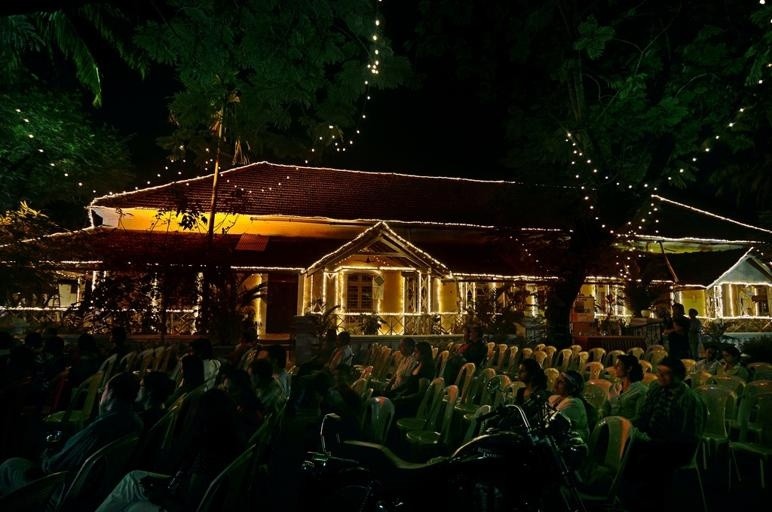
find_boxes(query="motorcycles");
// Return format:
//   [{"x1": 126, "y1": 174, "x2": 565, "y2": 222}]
[{"x1": 294, "y1": 395, "x2": 588, "y2": 511}]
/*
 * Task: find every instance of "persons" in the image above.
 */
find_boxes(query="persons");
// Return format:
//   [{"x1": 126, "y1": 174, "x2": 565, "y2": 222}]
[{"x1": 1, "y1": 297, "x2": 771, "y2": 512}]
